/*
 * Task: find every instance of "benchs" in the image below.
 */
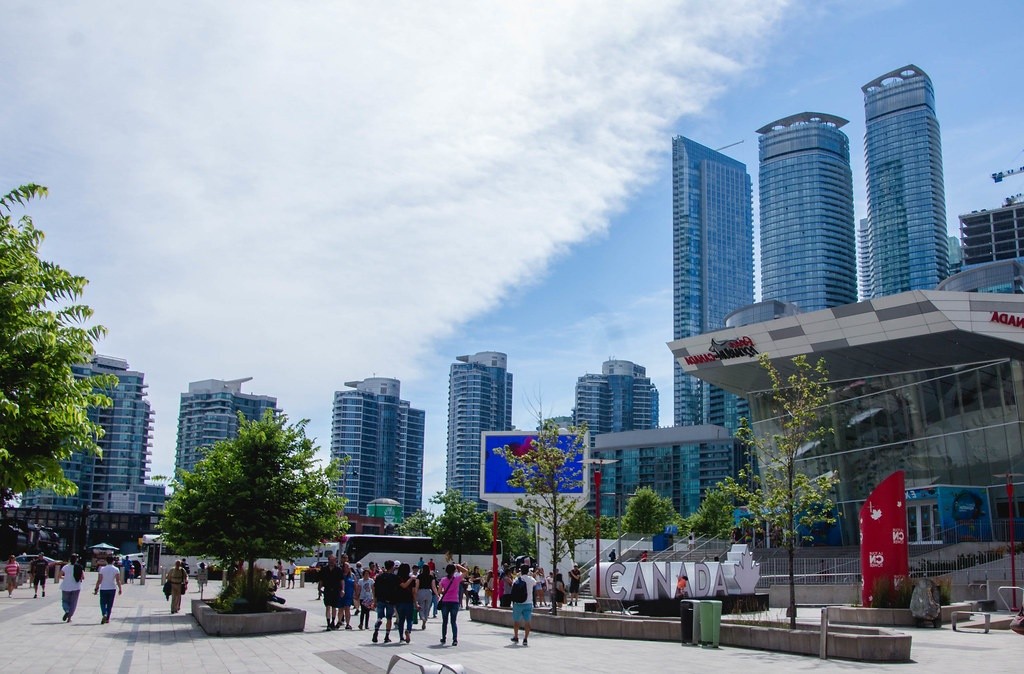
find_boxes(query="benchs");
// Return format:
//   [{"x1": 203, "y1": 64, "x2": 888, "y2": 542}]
[
  {"x1": 950, "y1": 610, "x2": 990, "y2": 634},
  {"x1": 596, "y1": 598, "x2": 639, "y2": 616},
  {"x1": 387, "y1": 653, "x2": 466, "y2": 674}
]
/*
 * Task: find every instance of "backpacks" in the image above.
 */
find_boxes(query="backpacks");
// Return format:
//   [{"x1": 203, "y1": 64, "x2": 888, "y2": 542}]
[{"x1": 511, "y1": 576, "x2": 527, "y2": 603}]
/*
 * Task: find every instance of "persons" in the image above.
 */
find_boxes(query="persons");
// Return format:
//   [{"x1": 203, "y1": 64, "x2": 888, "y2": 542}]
[
  {"x1": 336, "y1": 555, "x2": 565, "y2": 630},
  {"x1": 567, "y1": 563, "x2": 581, "y2": 606},
  {"x1": 318, "y1": 554, "x2": 344, "y2": 631},
  {"x1": 675, "y1": 575, "x2": 688, "y2": 598},
  {"x1": 688, "y1": 528, "x2": 695, "y2": 550},
  {"x1": 394, "y1": 563, "x2": 416, "y2": 643},
  {"x1": 167, "y1": 560, "x2": 188, "y2": 614},
  {"x1": 274, "y1": 559, "x2": 297, "y2": 589},
  {"x1": 511, "y1": 565, "x2": 537, "y2": 645},
  {"x1": 5, "y1": 555, "x2": 20, "y2": 598},
  {"x1": 113, "y1": 556, "x2": 135, "y2": 584},
  {"x1": 415, "y1": 564, "x2": 439, "y2": 629},
  {"x1": 608, "y1": 548, "x2": 616, "y2": 562},
  {"x1": 261, "y1": 570, "x2": 286, "y2": 604},
  {"x1": 60, "y1": 553, "x2": 84, "y2": 622},
  {"x1": 33, "y1": 552, "x2": 48, "y2": 598},
  {"x1": 372, "y1": 560, "x2": 417, "y2": 643},
  {"x1": 642, "y1": 551, "x2": 647, "y2": 562},
  {"x1": 140, "y1": 562, "x2": 147, "y2": 585},
  {"x1": 94, "y1": 556, "x2": 122, "y2": 624},
  {"x1": 438, "y1": 564, "x2": 468, "y2": 645}
]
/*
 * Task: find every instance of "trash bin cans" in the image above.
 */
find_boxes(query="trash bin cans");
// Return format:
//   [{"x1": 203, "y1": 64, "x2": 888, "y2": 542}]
[
  {"x1": 699, "y1": 600, "x2": 722, "y2": 647},
  {"x1": 680, "y1": 599, "x2": 701, "y2": 645}
]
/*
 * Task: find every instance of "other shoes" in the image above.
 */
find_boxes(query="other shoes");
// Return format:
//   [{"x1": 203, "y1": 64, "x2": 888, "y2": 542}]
[
  {"x1": 568, "y1": 601, "x2": 572, "y2": 605},
  {"x1": 452, "y1": 640, "x2": 457, "y2": 645},
  {"x1": 171, "y1": 609, "x2": 177, "y2": 614},
  {"x1": 405, "y1": 630, "x2": 410, "y2": 643},
  {"x1": 373, "y1": 633, "x2": 377, "y2": 642},
  {"x1": 327, "y1": 622, "x2": 335, "y2": 630},
  {"x1": 63, "y1": 610, "x2": 70, "y2": 622},
  {"x1": 523, "y1": 639, "x2": 527, "y2": 645},
  {"x1": 34, "y1": 595, "x2": 37, "y2": 598},
  {"x1": 400, "y1": 637, "x2": 406, "y2": 642},
  {"x1": 42, "y1": 592, "x2": 45, "y2": 597},
  {"x1": 355, "y1": 610, "x2": 359, "y2": 615},
  {"x1": 422, "y1": 625, "x2": 426, "y2": 630},
  {"x1": 336, "y1": 623, "x2": 340, "y2": 628},
  {"x1": 394, "y1": 622, "x2": 399, "y2": 629},
  {"x1": 358, "y1": 625, "x2": 362, "y2": 630},
  {"x1": 441, "y1": 637, "x2": 446, "y2": 642},
  {"x1": 385, "y1": 637, "x2": 391, "y2": 642},
  {"x1": 102, "y1": 614, "x2": 108, "y2": 624},
  {"x1": 345, "y1": 625, "x2": 352, "y2": 629},
  {"x1": 511, "y1": 637, "x2": 518, "y2": 642}
]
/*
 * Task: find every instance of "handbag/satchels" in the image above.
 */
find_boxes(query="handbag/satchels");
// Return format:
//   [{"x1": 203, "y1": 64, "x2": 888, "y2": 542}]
[
  {"x1": 437, "y1": 601, "x2": 442, "y2": 610},
  {"x1": 361, "y1": 596, "x2": 372, "y2": 610},
  {"x1": 181, "y1": 581, "x2": 185, "y2": 594}
]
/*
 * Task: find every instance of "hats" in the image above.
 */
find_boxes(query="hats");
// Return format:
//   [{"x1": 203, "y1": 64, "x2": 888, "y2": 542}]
[
  {"x1": 10, "y1": 555, "x2": 15, "y2": 558},
  {"x1": 394, "y1": 560, "x2": 401, "y2": 568},
  {"x1": 573, "y1": 563, "x2": 578, "y2": 567}
]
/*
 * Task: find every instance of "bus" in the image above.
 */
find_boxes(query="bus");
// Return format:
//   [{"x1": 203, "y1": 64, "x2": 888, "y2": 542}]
[
  {"x1": 141, "y1": 533, "x2": 340, "y2": 581},
  {"x1": 340, "y1": 532, "x2": 502, "y2": 583}
]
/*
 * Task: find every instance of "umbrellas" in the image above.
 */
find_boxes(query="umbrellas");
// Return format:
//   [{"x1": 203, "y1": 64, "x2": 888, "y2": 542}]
[{"x1": 89, "y1": 543, "x2": 119, "y2": 556}]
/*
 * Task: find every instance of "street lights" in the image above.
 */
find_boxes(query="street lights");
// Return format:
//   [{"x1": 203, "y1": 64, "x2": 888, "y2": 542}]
[
  {"x1": 1006, "y1": 473, "x2": 1020, "y2": 611},
  {"x1": 593, "y1": 460, "x2": 605, "y2": 598},
  {"x1": 341, "y1": 452, "x2": 359, "y2": 517},
  {"x1": 492, "y1": 511, "x2": 498, "y2": 607}
]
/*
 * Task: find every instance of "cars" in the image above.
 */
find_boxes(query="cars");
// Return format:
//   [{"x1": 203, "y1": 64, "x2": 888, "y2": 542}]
[
  {"x1": 0, "y1": 555, "x2": 63, "y2": 577},
  {"x1": 117, "y1": 552, "x2": 143, "y2": 566},
  {"x1": 309, "y1": 560, "x2": 329, "y2": 571}
]
